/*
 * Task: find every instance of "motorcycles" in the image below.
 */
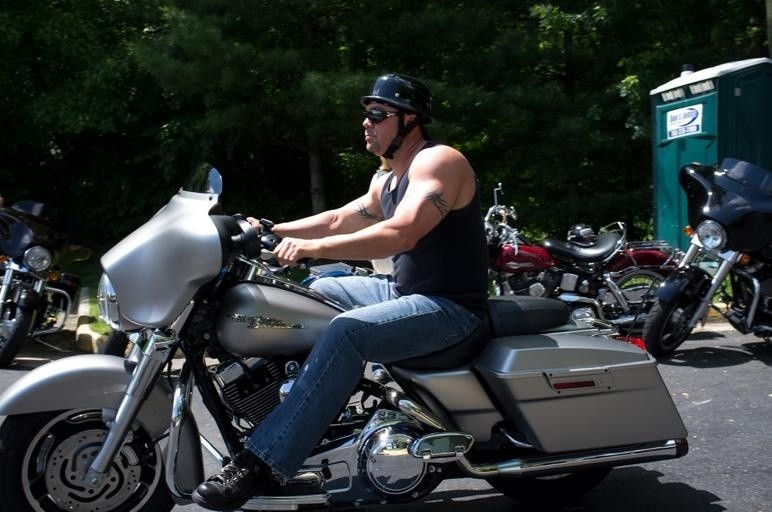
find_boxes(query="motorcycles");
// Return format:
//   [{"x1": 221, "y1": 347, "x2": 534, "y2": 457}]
[
  {"x1": 1, "y1": 166, "x2": 689, "y2": 512},
  {"x1": 483, "y1": 182, "x2": 684, "y2": 338},
  {"x1": 1, "y1": 198, "x2": 92, "y2": 371},
  {"x1": 642, "y1": 156, "x2": 771, "y2": 361}
]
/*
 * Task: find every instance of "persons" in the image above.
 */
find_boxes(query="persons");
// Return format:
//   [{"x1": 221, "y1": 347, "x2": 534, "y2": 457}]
[{"x1": 192, "y1": 73, "x2": 486, "y2": 512}]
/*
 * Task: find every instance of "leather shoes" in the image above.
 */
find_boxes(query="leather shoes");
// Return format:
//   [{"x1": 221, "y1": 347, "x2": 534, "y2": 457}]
[{"x1": 191, "y1": 458, "x2": 258, "y2": 511}]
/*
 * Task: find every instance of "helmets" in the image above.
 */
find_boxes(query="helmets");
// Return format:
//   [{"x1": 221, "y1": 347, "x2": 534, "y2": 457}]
[
  {"x1": 567, "y1": 223, "x2": 598, "y2": 247},
  {"x1": 359, "y1": 73, "x2": 433, "y2": 124}
]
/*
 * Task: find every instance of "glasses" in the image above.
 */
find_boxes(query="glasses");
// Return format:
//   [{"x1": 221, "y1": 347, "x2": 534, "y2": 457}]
[{"x1": 362, "y1": 107, "x2": 400, "y2": 123}]
